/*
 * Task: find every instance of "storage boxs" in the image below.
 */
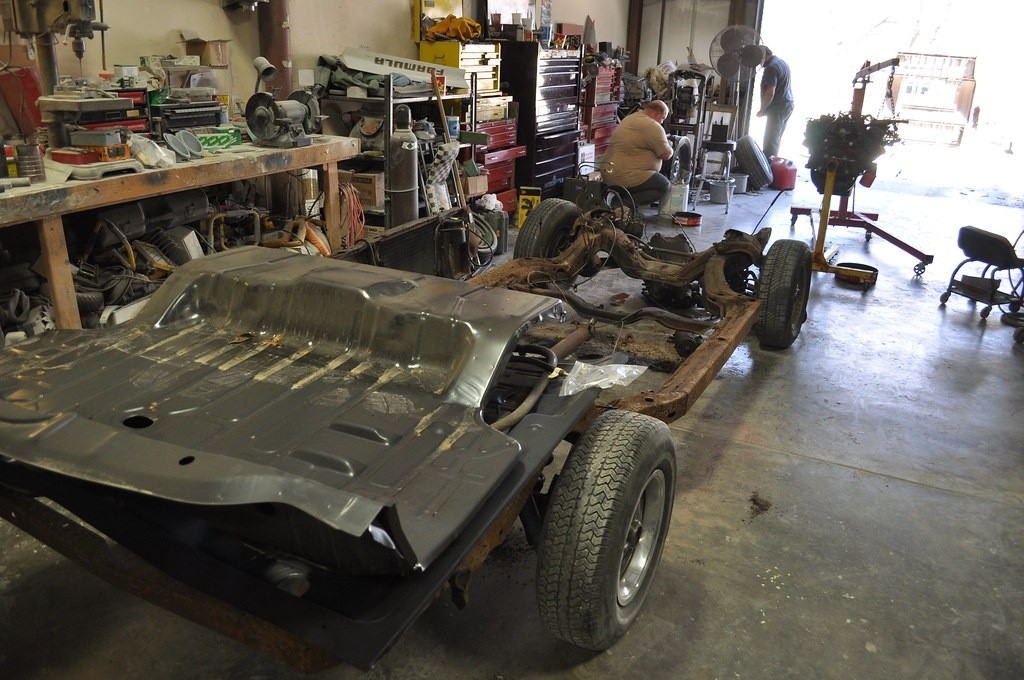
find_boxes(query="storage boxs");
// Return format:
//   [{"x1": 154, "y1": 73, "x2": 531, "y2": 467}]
[
  {"x1": 50, "y1": 149, "x2": 97, "y2": 165},
  {"x1": 160, "y1": 226, "x2": 203, "y2": 266},
  {"x1": 112, "y1": 64, "x2": 139, "y2": 81},
  {"x1": 70, "y1": 131, "x2": 121, "y2": 146},
  {"x1": 140, "y1": 54, "x2": 166, "y2": 66},
  {"x1": 176, "y1": 29, "x2": 234, "y2": 68},
  {"x1": 352, "y1": 170, "x2": 385, "y2": 207},
  {"x1": 460, "y1": 175, "x2": 489, "y2": 194},
  {"x1": 710, "y1": 123, "x2": 729, "y2": 142},
  {"x1": 411, "y1": 0, "x2": 501, "y2": 94}
]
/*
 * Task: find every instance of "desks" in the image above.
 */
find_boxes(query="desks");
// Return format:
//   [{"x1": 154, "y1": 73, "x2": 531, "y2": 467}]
[{"x1": 0, "y1": 124, "x2": 359, "y2": 331}]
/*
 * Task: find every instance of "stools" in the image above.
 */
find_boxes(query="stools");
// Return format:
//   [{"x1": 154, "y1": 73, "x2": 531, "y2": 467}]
[{"x1": 693, "y1": 139, "x2": 737, "y2": 214}]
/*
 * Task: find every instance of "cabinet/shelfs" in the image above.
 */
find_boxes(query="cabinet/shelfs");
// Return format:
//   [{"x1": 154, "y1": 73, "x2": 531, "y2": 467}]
[
  {"x1": 502, "y1": 41, "x2": 584, "y2": 199},
  {"x1": 317, "y1": 72, "x2": 478, "y2": 231}
]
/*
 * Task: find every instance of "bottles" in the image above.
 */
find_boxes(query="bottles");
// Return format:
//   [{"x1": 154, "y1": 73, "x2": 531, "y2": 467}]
[
  {"x1": 517, "y1": 26, "x2": 524, "y2": 42},
  {"x1": 3, "y1": 144, "x2": 17, "y2": 177}
]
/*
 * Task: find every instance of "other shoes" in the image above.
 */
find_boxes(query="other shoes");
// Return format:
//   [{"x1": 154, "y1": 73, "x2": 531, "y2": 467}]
[{"x1": 618, "y1": 199, "x2": 643, "y2": 217}]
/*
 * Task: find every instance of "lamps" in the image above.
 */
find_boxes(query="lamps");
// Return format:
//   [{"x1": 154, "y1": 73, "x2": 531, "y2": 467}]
[{"x1": 254, "y1": 56, "x2": 278, "y2": 93}]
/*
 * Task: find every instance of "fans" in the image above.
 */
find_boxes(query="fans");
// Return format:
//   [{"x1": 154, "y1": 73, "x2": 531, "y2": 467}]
[{"x1": 708, "y1": 23, "x2": 766, "y2": 172}]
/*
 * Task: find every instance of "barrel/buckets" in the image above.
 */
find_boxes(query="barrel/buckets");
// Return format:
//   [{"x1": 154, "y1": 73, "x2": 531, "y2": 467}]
[
  {"x1": 660, "y1": 179, "x2": 689, "y2": 214},
  {"x1": 710, "y1": 182, "x2": 736, "y2": 204},
  {"x1": 729, "y1": 173, "x2": 749, "y2": 193},
  {"x1": 770, "y1": 157, "x2": 797, "y2": 190}
]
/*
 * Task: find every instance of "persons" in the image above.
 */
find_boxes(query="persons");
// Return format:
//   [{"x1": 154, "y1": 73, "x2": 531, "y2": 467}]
[
  {"x1": 600, "y1": 101, "x2": 674, "y2": 205},
  {"x1": 756, "y1": 45, "x2": 794, "y2": 165}
]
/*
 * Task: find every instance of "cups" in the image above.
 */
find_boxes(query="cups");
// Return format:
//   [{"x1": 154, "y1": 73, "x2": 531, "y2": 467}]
[
  {"x1": 541, "y1": 40, "x2": 551, "y2": 48},
  {"x1": 14, "y1": 144, "x2": 46, "y2": 183},
  {"x1": 522, "y1": 17, "x2": 533, "y2": 30},
  {"x1": 541, "y1": 24, "x2": 552, "y2": 39},
  {"x1": 448, "y1": 116, "x2": 459, "y2": 136},
  {"x1": 491, "y1": 13, "x2": 501, "y2": 31},
  {"x1": 512, "y1": 13, "x2": 522, "y2": 25}
]
